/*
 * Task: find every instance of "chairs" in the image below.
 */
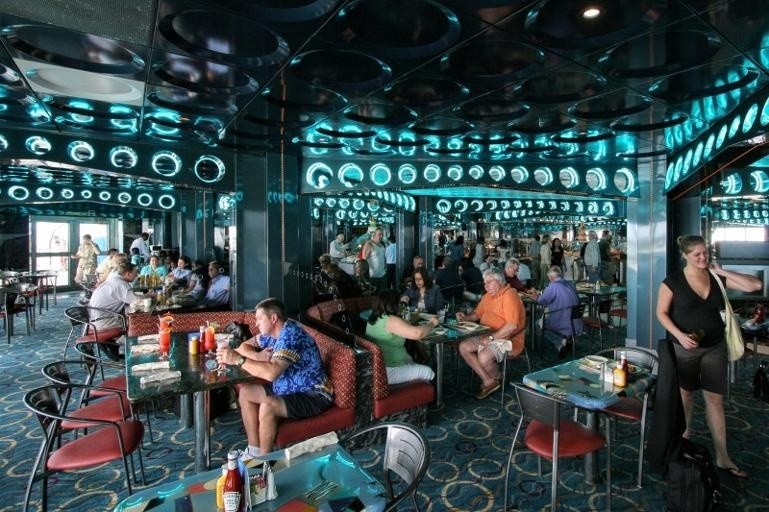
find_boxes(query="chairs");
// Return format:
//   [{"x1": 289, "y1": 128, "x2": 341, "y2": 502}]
[
  {"x1": 402, "y1": 259, "x2": 769, "y2": 512},
  {"x1": 0, "y1": 266, "x2": 200, "y2": 511},
  {"x1": 342, "y1": 422, "x2": 428, "y2": 511}
]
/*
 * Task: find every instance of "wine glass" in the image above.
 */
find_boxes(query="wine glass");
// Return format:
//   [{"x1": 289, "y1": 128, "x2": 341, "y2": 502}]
[
  {"x1": 158, "y1": 329, "x2": 171, "y2": 360},
  {"x1": 215, "y1": 335, "x2": 231, "y2": 374},
  {"x1": 203, "y1": 327, "x2": 215, "y2": 357}
]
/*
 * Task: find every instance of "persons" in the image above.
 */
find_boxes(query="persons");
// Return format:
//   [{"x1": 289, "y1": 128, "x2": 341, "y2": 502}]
[
  {"x1": 741, "y1": 303, "x2": 765, "y2": 328},
  {"x1": 654, "y1": 234, "x2": 763, "y2": 480},
  {"x1": 70, "y1": 232, "x2": 230, "y2": 362},
  {"x1": 313, "y1": 227, "x2": 627, "y2": 401},
  {"x1": 214, "y1": 296, "x2": 334, "y2": 459}
]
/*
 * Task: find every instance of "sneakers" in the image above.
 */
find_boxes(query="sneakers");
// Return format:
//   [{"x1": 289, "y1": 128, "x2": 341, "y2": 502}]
[
  {"x1": 100, "y1": 338, "x2": 120, "y2": 362},
  {"x1": 475, "y1": 373, "x2": 503, "y2": 400}
]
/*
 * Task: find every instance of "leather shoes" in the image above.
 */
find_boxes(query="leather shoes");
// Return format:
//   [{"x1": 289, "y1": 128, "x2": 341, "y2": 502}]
[
  {"x1": 558, "y1": 345, "x2": 568, "y2": 360},
  {"x1": 566, "y1": 339, "x2": 572, "y2": 351}
]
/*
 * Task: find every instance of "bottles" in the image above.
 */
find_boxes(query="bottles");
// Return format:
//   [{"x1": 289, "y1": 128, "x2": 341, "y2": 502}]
[
  {"x1": 596, "y1": 281, "x2": 601, "y2": 289},
  {"x1": 156, "y1": 286, "x2": 169, "y2": 311},
  {"x1": 614, "y1": 352, "x2": 628, "y2": 387},
  {"x1": 752, "y1": 303, "x2": 763, "y2": 323},
  {"x1": 216, "y1": 466, "x2": 228, "y2": 509},
  {"x1": 599, "y1": 362, "x2": 607, "y2": 380},
  {"x1": 599, "y1": 381, "x2": 605, "y2": 397},
  {"x1": 262, "y1": 459, "x2": 271, "y2": 481},
  {"x1": 199, "y1": 326, "x2": 206, "y2": 353},
  {"x1": 265, "y1": 464, "x2": 278, "y2": 501},
  {"x1": 190, "y1": 336, "x2": 199, "y2": 355},
  {"x1": 222, "y1": 450, "x2": 244, "y2": 512}
]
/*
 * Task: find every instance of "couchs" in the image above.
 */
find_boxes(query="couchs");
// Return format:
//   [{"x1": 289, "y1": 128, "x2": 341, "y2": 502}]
[
  {"x1": 304, "y1": 294, "x2": 437, "y2": 420},
  {"x1": 129, "y1": 310, "x2": 358, "y2": 450}
]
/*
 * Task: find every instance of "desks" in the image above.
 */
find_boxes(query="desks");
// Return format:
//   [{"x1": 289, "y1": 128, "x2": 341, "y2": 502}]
[
  {"x1": 123, "y1": 329, "x2": 260, "y2": 472},
  {"x1": 113, "y1": 441, "x2": 386, "y2": 511},
  {"x1": 356, "y1": 300, "x2": 494, "y2": 414}
]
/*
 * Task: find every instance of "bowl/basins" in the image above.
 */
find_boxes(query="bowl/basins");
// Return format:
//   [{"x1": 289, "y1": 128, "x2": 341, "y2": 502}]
[{"x1": 585, "y1": 353, "x2": 607, "y2": 367}]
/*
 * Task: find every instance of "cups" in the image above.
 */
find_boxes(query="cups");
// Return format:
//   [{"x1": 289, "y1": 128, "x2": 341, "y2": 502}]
[
  {"x1": 144, "y1": 307, "x2": 152, "y2": 312},
  {"x1": 160, "y1": 318, "x2": 172, "y2": 329},
  {"x1": 144, "y1": 298, "x2": 153, "y2": 307}
]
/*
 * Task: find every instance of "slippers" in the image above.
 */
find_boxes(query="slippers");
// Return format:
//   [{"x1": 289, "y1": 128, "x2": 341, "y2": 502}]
[{"x1": 716, "y1": 465, "x2": 746, "y2": 478}]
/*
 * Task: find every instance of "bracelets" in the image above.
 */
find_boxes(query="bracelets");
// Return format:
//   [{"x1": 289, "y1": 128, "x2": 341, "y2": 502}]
[{"x1": 237, "y1": 356, "x2": 245, "y2": 367}]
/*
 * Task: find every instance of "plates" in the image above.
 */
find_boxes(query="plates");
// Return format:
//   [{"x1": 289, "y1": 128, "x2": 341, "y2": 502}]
[
  {"x1": 609, "y1": 362, "x2": 642, "y2": 373},
  {"x1": 432, "y1": 328, "x2": 448, "y2": 335},
  {"x1": 455, "y1": 321, "x2": 479, "y2": 330}
]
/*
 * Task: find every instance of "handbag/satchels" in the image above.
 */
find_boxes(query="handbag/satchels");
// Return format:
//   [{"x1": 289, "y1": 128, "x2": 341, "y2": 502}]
[
  {"x1": 724, "y1": 303, "x2": 745, "y2": 362},
  {"x1": 661, "y1": 435, "x2": 713, "y2": 512}
]
